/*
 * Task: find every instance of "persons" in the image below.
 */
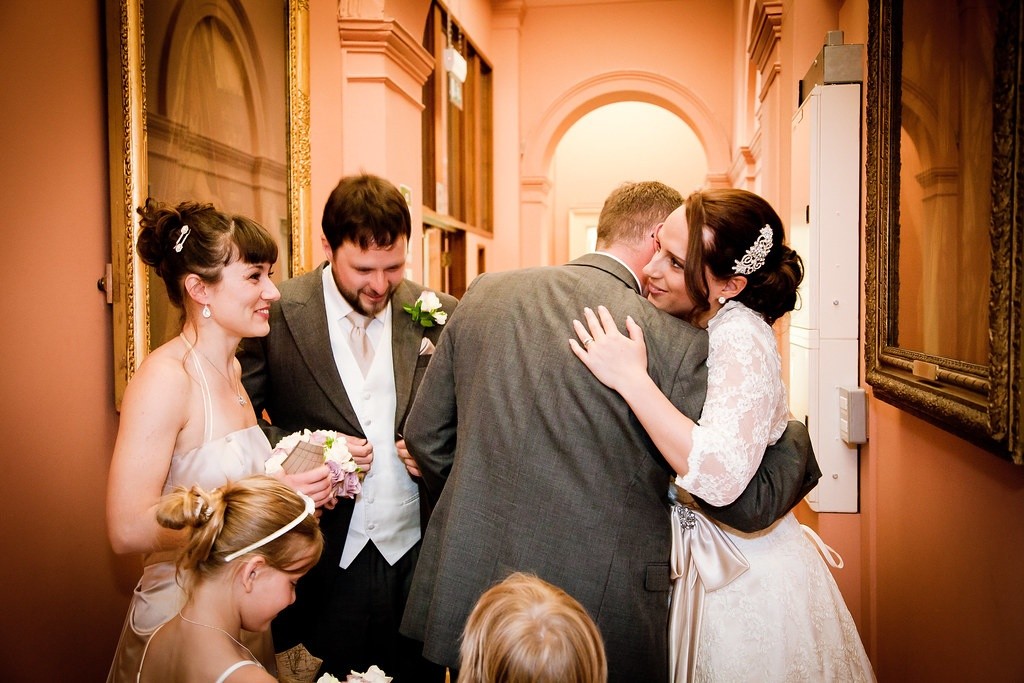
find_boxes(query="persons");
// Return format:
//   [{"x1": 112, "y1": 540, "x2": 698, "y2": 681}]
[
  {"x1": 399, "y1": 181, "x2": 822, "y2": 683},
  {"x1": 568, "y1": 187, "x2": 877, "y2": 683},
  {"x1": 233, "y1": 176, "x2": 461, "y2": 683},
  {"x1": 457, "y1": 573, "x2": 608, "y2": 682},
  {"x1": 108, "y1": 196, "x2": 332, "y2": 683},
  {"x1": 137, "y1": 475, "x2": 323, "y2": 682}
]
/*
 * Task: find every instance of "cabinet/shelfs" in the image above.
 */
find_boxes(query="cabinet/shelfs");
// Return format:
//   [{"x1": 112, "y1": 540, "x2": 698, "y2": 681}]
[{"x1": 790, "y1": 83, "x2": 862, "y2": 512}]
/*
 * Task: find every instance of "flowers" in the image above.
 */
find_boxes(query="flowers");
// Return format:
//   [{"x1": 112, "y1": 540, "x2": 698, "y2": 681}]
[
  {"x1": 317, "y1": 665, "x2": 394, "y2": 683},
  {"x1": 266, "y1": 426, "x2": 362, "y2": 501},
  {"x1": 400, "y1": 288, "x2": 448, "y2": 328}
]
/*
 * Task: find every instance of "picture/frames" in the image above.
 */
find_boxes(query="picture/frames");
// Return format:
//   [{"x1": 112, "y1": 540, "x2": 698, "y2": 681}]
[
  {"x1": 105, "y1": 0, "x2": 314, "y2": 410},
  {"x1": 864, "y1": 0, "x2": 1024, "y2": 468}
]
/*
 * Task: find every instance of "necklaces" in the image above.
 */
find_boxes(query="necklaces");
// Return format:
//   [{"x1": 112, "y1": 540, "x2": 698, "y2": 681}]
[
  {"x1": 183, "y1": 332, "x2": 247, "y2": 406},
  {"x1": 179, "y1": 611, "x2": 265, "y2": 669}
]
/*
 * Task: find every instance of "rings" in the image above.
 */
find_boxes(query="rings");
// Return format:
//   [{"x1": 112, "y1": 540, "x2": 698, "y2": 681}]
[{"x1": 584, "y1": 339, "x2": 594, "y2": 347}]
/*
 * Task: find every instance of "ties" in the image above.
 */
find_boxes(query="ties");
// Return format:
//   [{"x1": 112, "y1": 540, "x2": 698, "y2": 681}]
[{"x1": 345, "y1": 311, "x2": 373, "y2": 378}]
